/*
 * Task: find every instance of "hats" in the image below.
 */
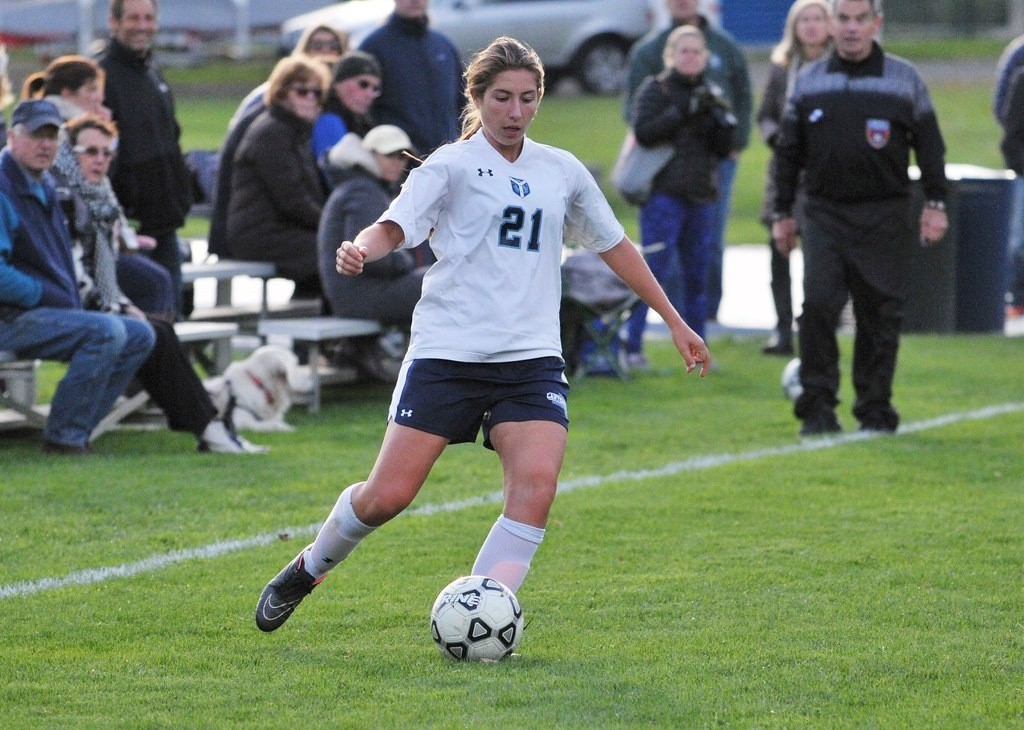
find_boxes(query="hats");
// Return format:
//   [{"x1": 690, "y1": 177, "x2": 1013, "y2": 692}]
[
  {"x1": 360, "y1": 123, "x2": 417, "y2": 156},
  {"x1": 11, "y1": 99, "x2": 62, "y2": 134},
  {"x1": 333, "y1": 50, "x2": 384, "y2": 83}
]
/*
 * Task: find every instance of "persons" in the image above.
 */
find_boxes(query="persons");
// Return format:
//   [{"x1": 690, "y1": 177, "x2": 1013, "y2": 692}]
[
  {"x1": 621, "y1": 1, "x2": 751, "y2": 369},
  {"x1": 91, "y1": 0, "x2": 196, "y2": 309},
  {"x1": 315, "y1": 124, "x2": 436, "y2": 324},
  {"x1": 992, "y1": 31, "x2": 1024, "y2": 338},
  {"x1": 762, "y1": 0, "x2": 951, "y2": 438},
  {"x1": 254, "y1": 37, "x2": 712, "y2": 646},
  {"x1": 19, "y1": 54, "x2": 175, "y2": 320},
  {"x1": 0, "y1": 97, "x2": 252, "y2": 463},
  {"x1": 209, "y1": 0, "x2": 468, "y2": 356},
  {"x1": 755, "y1": 0, "x2": 831, "y2": 356}
]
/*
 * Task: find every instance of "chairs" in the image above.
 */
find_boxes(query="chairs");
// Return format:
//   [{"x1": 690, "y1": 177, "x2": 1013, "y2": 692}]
[{"x1": 560, "y1": 241, "x2": 666, "y2": 382}]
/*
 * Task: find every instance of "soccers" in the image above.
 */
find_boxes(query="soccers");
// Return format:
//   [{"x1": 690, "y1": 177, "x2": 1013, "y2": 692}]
[{"x1": 430, "y1": 574, "x2": 525, "y2": 663}]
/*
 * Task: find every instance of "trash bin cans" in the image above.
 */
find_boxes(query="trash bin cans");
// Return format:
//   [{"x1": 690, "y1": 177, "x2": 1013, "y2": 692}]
[{"x1": 903, "y1": 163, "x2": 1016, "y2": 333}]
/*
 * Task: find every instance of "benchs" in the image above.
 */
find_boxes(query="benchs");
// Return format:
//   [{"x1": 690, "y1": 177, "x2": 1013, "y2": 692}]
[{"x1": 0, "y1": 258, "x2": 380, "y2": 433}]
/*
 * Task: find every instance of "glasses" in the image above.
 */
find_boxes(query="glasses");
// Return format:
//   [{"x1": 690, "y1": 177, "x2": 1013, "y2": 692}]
[
  {"x1": 286, "y1": 84, "x2": 322, "y2": 98},
  {"x1": 308, "y1": 38, "x2": 341, "y2": 51},
  {"x1": 71, "y1": 144, "x2": 116, "y2": 158}
]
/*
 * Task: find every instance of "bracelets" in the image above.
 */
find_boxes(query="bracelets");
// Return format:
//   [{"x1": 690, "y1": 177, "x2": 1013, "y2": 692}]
[{"x1": 924, "y1": 198, "x2": 945, "y2": 210}]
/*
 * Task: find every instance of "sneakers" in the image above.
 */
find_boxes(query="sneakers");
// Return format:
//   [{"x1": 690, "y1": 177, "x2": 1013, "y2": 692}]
[
  {"x1": 347, "y1": 336, "x2": 401, "y2": 385},
  {"x1": 252, "y1": 541, "x2": 331, "y2": 633},
  {"x1": 196, "y1": 433, "x2": 268, "y2": 456}
]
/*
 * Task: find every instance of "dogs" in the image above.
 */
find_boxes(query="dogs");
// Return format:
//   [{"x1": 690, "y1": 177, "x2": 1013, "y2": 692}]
[{"x1": 200, "y1": 344, "x2": 315, "y2": 434}]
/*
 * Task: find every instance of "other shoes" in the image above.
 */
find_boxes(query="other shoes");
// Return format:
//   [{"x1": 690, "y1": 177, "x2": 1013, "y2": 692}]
[
  {"x1": 856, "y1": 418, "x2": 899, "y2": 435},
  {"x1": 628, "y1": 352, "x2": 646, "y2": 370},
  {"x1": 800, "y1": 417, "x2": 842, "y2": 441},
  {"x1": 761, "y1": 331, "x2": 794, "y2": 357}
]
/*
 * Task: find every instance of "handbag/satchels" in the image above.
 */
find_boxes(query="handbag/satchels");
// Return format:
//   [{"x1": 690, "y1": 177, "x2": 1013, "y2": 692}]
[{"x1": 611, "y1": 127, "x2": 677, "y2": 207}]
